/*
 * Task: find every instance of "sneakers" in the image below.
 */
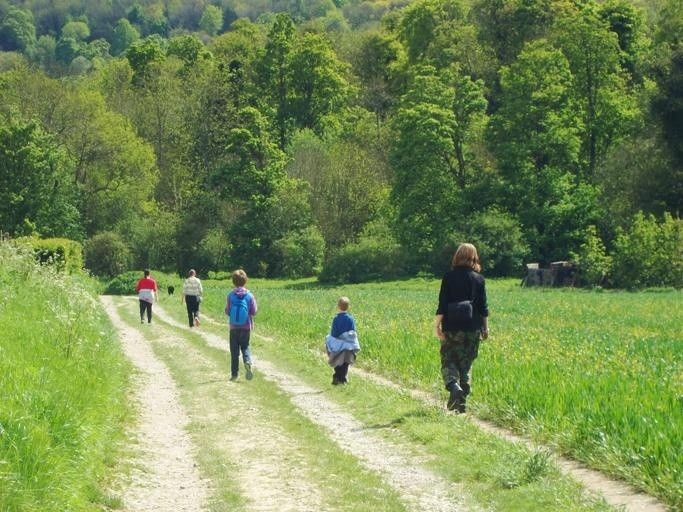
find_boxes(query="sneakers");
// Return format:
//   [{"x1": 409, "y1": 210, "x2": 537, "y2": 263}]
[
  {"x1": 195, "y1": 317, "x2": 200, "y2": 327},
  {"x1": 229, "y1": 361, "x2": 253, "y2": 383}
]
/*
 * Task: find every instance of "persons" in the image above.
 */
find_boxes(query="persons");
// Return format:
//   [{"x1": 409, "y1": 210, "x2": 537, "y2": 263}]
[
  {"x1": 135, "y1": 269, "x2": 158, "y2": 323},
  {"x1": 324, "y1": 297, "x2": 361, "y2": 385},
  {"x1": 180, "y1": 268, "x2": 203, "y2": 328},
  {"x1": 223, "y1": 269, "x2": 256, "y2": 381},
  {"x1": 434, "y1": 242, "x2": 489, "y2": 413}
]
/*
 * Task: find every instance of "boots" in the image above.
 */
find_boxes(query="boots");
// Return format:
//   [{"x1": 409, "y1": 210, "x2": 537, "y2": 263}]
[{"x1": 447, "y1": 382, "x2": 469, "y2": 413}]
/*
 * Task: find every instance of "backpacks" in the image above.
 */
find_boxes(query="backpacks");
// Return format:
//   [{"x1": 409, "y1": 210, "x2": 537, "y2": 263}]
[{"x1": 228, "y1": 291, "x2": 252, "y2": 326}]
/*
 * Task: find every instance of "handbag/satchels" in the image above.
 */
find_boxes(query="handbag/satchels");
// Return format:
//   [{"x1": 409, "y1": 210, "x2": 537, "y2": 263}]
[
  {"x1": 446, "y1": 300, "x2": 473, "y2": 325},
  {"x1": 196, "y1": 295, "x2": 202, "y2": 303}
]
